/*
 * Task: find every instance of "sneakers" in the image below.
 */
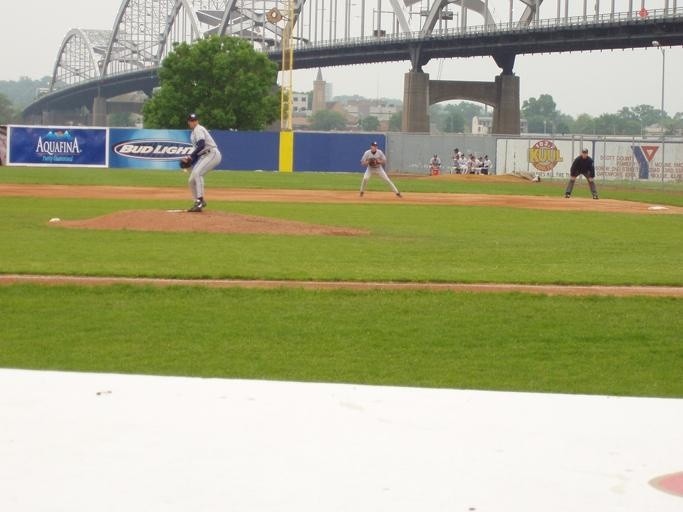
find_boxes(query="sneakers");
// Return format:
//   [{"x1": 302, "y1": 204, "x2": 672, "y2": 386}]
[
  {"x1": 565, "y1": 192, "x2": 570, "y2": 198},
  {"x1": 592, "y1": 194, "x2": 598, "y2": 199},
  {"x1": 187, "y1": 198, "x2": 206, "y2": 212}
]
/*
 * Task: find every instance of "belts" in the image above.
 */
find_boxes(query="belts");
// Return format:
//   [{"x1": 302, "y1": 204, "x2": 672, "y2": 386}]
[{"x1": 198, "y1": 150, "x2": 210, "y2": 156}]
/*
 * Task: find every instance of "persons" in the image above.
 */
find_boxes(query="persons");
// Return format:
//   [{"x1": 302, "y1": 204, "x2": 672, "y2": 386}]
[
  {"x1": 360, "y1": 141, "x2": 402, "y2": 198},
  {"x1": 429, "y1": 153, "x2": 441, "y2": 176},
  {"x1": 453, "y1": 148, "x2": 492, "y2": 175},
  {"x1": 180, "y1": 113, "x2": 222, "y2": 212},
  {"x1": 565, "y1": 147, "x2": 599, "y2": 199}
]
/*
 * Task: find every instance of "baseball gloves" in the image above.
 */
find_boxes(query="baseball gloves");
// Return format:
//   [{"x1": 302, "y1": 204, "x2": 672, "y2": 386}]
[
  {"x1": 180, "y1": 156, "x2": 192, "y2": 167},
  {"x1": 369, "y1": 158, "x2": 378, "y2": 166}
]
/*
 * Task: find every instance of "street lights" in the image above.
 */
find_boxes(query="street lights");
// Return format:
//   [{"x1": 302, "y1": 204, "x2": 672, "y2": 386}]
[{"x1": 649, "y1": 39, "x2": 666, "y2": 184}]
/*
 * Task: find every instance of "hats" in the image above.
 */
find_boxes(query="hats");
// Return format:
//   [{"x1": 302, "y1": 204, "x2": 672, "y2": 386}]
[
  {"x1": 582, "y1": 148, "x2": 588, "y2": 154},
  {"x1": 187, "y1": 113, "x2": 198, "y2": 121},
  {"x1": 371, "y1": 141, "x2": 377, "y2": 146}
]
